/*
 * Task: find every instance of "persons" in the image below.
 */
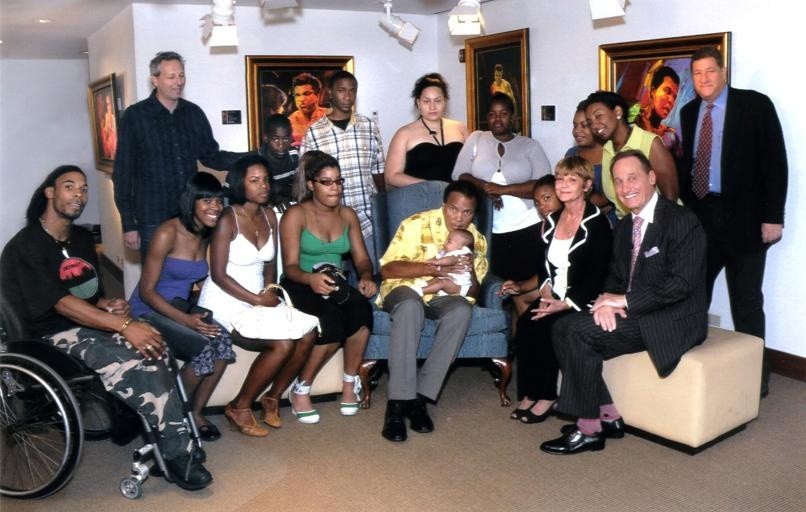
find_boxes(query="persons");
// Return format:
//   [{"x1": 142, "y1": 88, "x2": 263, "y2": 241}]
[
  {"x1": 126, "y1": 168, "x2": 236, "y2": 444},
  {"x1": 678, "y1": 46, "x2": 790, "y2": 399},
  {"x1": 104, "y1": 95, "x2": 116, "y2": 134},
  {"x1": 198, "y1": 157, "x2": 321, "y2": 436},
  {"x1": 1, "y1": 164, "x2": 216, "y2": 493},
  {"x1": 540, "y1": 149, "x2": 713, "y2": 455},
  {"x1": 225, "y1": 64, "x2": 678, "y2": 299},
  {"x1": 279, "y1": 151, "x2": 378, "y2": 423},
  {"x1": 109, "y1": 50, "x2": 254, "y2": 274},
  {"x1": 512, "y1": 156, "x2": 613, "y2": 423},
  {"x1": 375, "y1": 181, "x2": 488, "y2": 442},
  {"x1": 497, "y1": 175, "x2": 563, "y2": 317}
]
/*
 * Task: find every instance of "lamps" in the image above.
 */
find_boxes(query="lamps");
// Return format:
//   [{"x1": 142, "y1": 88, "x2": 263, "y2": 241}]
[
  {"x1": 446, "y1": 0, "x2": 484, "y2": 38},
  {"x1": 378, "y1": 0, "x2": 419, "y2": 46},
  {"x1": 200, "y1": 0, "x2": 239, "y2": 50}
]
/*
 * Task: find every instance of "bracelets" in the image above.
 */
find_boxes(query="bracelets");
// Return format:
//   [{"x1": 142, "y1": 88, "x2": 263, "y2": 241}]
[{"x1": 118, "y1": 317, "x2": 133, "y2": 336}]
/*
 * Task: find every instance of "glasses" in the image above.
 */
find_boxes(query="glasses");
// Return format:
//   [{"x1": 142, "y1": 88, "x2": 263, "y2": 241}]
[
  {"x1": 271, "y1": 138, "x2": 290, "y2": 143},
  {"x1": 314, "y1": 178, "x2": 345, "y2": 186}
]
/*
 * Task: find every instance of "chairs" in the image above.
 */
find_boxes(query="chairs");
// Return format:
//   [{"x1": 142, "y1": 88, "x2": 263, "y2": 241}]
[{"x1": 357, "y1": 177, "x2": 511, "y2": 408}]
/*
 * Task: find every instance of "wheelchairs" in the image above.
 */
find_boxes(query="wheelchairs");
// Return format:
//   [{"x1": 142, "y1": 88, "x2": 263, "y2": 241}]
[{"x1": 1, "y1": 321, "x2": 208, "y2": 501}]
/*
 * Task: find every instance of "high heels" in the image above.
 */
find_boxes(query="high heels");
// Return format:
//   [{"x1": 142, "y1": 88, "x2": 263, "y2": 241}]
[
  {"x1": 260, "y1": 393, "x2": 281, "y2": 428},
  {"x1": 520, "y1": 396, "x2": 559, "y2": 424},
  {"x1": 340, "y1": 372, "x2": 363, "y2": 416},
  {"x1": 288, "y1": 380, "x2": 321, "y2": 424},
  {"x1": 510, "y1": 401, "x2": 538, "y2": 419},
  {"x1": 224, "y1": 402, "x2": 269, "y2": 437}
]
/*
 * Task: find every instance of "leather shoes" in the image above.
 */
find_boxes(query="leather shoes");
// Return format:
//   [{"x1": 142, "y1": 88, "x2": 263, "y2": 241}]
[
  {"x1": 198, "y1": 425, "x2": 221, "y2": 441},
  {"x1": 406, "y1": 399, "x2": 433, "y2": 433},
  {"x1": 382, "y1": 400, "x2": 407, "y2": 441},
  {"x1": 163, "y1": 450, "x2": 211, "y2": 490},
  {"x1": 149, "y1": 447, "x2": 206, "y2": 476},
  {"x1": 540, "y1": 424, "x2": 605, "y2": 455},
  {"x1": 561, "y1": 417, "x2": 624, "y2": 438}
]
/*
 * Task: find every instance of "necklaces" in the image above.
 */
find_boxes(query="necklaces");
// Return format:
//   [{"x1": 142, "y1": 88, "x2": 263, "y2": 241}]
[{"x1": 38, "y1": 217, "x2": 71, "y2": 260}]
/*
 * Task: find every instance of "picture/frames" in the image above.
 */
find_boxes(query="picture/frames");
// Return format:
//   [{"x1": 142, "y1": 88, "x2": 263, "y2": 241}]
[
  {"x1": 248, "y1": 50, "x2": 356, "y2": 166},
  {"x1": 87, "y1": 72, "x2": 124, "y2": 175},
  {"x1": 464, "y1": 27, "x2": 533, "y2": 142},
  {"x1": 598, "y1": 32, "x2": 731, "y2": 153}
]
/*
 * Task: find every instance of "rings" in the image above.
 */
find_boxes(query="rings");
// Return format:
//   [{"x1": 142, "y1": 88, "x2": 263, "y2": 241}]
[{"x1": 143, "y1": 344, "x2": 153, "y2": 352}]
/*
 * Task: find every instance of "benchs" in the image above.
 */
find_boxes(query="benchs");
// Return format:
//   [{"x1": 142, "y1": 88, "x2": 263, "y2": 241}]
[
  {"x1": 176, "y1": 342, "x2": 345, "y2": 411},
  {"x1": 553, "y1": 325, "x2": 766, "y2": 446}
]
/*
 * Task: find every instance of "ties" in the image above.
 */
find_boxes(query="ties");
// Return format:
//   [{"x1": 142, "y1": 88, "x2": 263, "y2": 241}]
[
  {"x1": 691, "y1": 105, "x2": 715, "y2": 200},
  {"x1": 631, "y1": 217, "x2": 644, "y2": 267}
]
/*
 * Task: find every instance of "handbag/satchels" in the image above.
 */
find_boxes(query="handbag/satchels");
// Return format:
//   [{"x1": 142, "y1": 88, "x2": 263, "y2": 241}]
[
  {"x1": 312, "y1": 262, "x2": 350, "y2": 305},
  {"x1": 171, "y1": 297, "x2": 212, "y2": 325}
]
